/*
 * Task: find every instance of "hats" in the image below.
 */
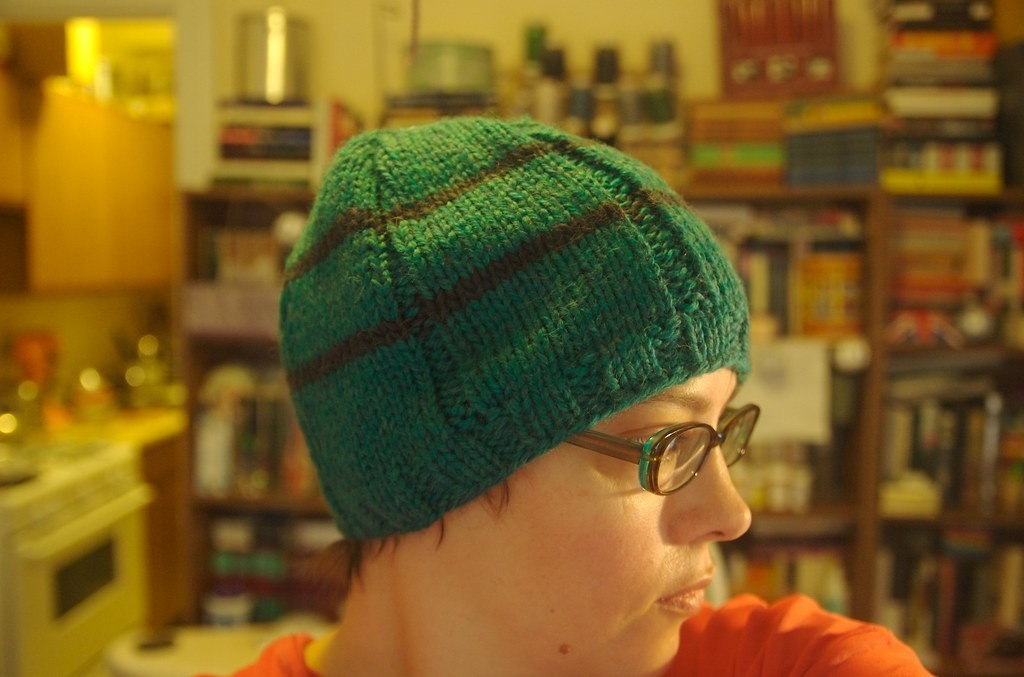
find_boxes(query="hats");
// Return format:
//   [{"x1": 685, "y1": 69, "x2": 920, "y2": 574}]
[{"x1": 277, "y1": 114, "x2": 752, "y2": 546}]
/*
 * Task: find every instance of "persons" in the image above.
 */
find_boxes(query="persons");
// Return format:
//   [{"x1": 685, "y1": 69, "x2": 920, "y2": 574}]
[{"x1": 181, "y1": 117, "x2": 932, "y2": 677}]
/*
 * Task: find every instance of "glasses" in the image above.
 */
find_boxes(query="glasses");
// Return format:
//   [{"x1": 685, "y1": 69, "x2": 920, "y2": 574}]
[{"x1": 562, "y1": 402, "x2": 762, "y2": 497}]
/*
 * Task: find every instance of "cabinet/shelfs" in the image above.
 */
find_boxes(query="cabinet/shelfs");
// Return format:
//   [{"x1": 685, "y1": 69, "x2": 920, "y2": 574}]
[
  {"x1": 866, "y1": 190, "x2": 1024, "y2": 677},
  {"x1": 173, "y1": 183, "x2": 881, "y2": 619}
]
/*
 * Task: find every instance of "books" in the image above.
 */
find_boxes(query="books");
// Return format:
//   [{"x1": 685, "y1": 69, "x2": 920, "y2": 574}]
[
  {"x1": 194, "y1": 95, "x2": 523, "y2": 623},
  {"x1": 616, "y1": 0, "x2": 1023, "y2": 677}
]
